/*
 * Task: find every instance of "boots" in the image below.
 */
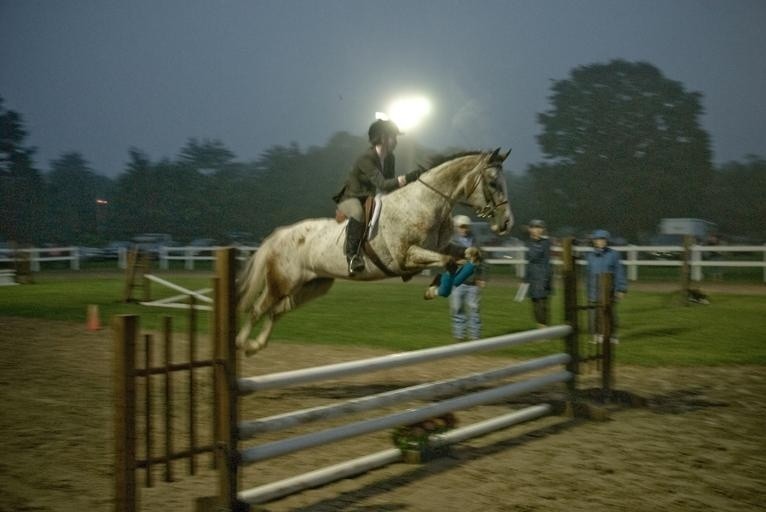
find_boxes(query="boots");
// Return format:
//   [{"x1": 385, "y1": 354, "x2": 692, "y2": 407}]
[{"x1": 343, "y1": 217, "x2": 366, "y2": 279}]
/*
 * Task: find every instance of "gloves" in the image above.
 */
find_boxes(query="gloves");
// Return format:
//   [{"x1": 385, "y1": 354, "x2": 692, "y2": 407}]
[{"x1": 405, "y1": 170, "x2": 421, "y2": 183}]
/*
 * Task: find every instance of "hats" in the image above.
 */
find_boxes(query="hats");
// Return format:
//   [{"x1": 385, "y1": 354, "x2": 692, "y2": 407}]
[
  {"x1": 452, "y1": 215, "x2": 474, "y2": 228},
  {"x1": 528, "y1": 219, "x2": 547, "y2": 229},
  {"x1": 368, "y1": 119, "x2": 407, "y2": 145},
  {"x1": 589, "y1": 229, "x2": 611, "y2": 240}
]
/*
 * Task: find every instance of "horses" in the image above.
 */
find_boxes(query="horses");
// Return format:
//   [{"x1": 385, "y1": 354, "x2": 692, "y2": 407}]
[{"x1": 233, "y1": 145, "x2": 514, "y2": 358}]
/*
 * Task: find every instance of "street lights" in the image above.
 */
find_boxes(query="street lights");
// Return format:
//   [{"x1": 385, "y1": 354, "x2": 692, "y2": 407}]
[{"x1": 384, "y1": 90, "x2": 436, "y2": 179}]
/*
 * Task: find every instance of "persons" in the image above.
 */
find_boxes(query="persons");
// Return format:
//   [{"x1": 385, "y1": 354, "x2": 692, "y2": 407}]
[
  {"x1": 525, "y1": 219, "x2": 552, "y2": 328},
  {"x1": 445, "y1": 214, "x2": 483, "y2": 340},
  {"x1": 585, "y1": 229, "x2": 626, "y2": 345},
  {"x1": 332, "y1": 120, "x2": 420, "y2": 275}
]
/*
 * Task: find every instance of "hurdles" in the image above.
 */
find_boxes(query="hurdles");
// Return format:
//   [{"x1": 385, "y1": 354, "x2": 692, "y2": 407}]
[{"x1": 111, "y1": 240, "x2": 615, "y2": 512}]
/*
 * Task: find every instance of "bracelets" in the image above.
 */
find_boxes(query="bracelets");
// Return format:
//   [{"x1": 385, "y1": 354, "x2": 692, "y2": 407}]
[{"x1": 398, "y1": 175, "x2": 406, "y2": 186}]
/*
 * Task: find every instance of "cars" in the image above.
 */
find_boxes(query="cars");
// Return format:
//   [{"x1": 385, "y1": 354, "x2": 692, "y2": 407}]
[{"x1": 80, "y1": 232, "x2": 257, "y2": 270}]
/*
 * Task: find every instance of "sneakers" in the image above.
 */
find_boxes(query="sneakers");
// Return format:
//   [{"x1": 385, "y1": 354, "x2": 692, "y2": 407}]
[{"x1": 593, "y1": 334, "x2": 620, "y2": 346}]
